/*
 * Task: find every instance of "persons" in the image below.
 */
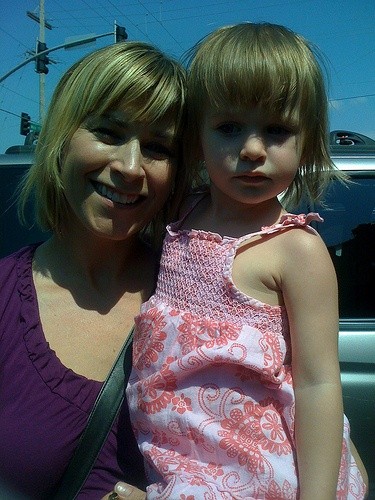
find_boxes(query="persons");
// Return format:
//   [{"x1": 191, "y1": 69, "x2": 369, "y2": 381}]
[
  {"x1": 125, "y1": 22, "x2": 369, "y2": 499},
  {"x1": 0, "y1": 42, "x2": 190, "y2": 499}
]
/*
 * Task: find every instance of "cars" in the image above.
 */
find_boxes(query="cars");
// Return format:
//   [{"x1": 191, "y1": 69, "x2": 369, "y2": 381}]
[
  {"x1": 1, "y1": 154, "x2": 375, "y2": 500},
  {"x1": 326, "y1": 130, "x2": 375, "y2": 155}
]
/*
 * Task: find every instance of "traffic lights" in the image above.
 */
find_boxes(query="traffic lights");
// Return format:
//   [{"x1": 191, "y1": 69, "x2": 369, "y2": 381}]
[
  {"x1": 20, "y1": 112, "x2": 32, "y2": 134},
  {"x1": 113, "y1": 19, "x2": 129, "y2": 45}
]
